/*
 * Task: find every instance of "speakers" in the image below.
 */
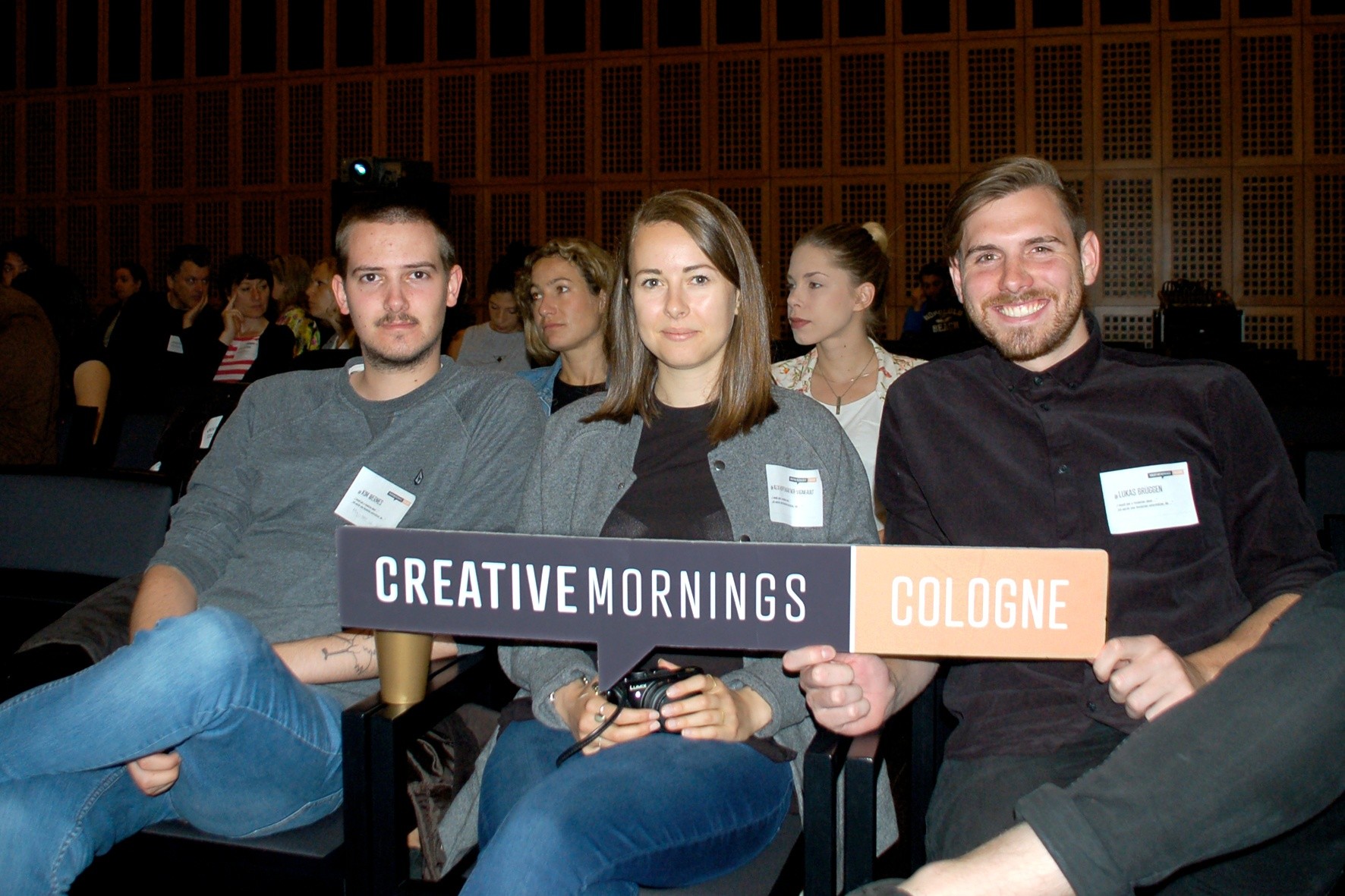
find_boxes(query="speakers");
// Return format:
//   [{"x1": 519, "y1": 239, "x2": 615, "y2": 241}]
[{"x1": 1152, "y1": 306, "x2": 1244, "y2": 362}]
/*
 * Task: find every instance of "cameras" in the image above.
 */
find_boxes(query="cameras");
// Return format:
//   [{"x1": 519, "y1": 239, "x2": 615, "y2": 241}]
[{"x1": 607, "y1": 664, "x2": 705, "y2": 731}]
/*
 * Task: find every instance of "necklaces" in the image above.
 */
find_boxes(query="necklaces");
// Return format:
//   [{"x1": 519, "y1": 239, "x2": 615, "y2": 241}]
[{"x1": 816, "y1": 349, "x2": 878, "y2": 415}]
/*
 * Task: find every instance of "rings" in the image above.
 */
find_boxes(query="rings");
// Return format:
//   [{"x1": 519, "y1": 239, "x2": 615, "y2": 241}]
[
  {"x1": 594, "y1": 702, "x2": 610, "y2": 723},
  {"x1": 702, "y1": 674, "x2": 716, "y2": 694},
  {"x1": 597, "y1": 737, "x2": 602, "y2": 748}
]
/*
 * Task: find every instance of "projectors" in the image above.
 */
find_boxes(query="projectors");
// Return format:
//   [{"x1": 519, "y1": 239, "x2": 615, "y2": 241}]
[{"x1": 339, "y1": 155, "x2": 380, "y2": 188}]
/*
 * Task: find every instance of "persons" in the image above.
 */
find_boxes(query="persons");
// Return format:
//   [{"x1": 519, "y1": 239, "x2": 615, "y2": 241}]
[
  {"x1": 0, "y1": 178, "x2": 550, "y2": 896},
  {"x1": 0, "y1": 238, "x2": 365, "y2": 490},
  {"x1": 779, "y1": 154, "x2": 1345, "y2": 896},
  {"x1": 899, "y1": 261, "x2": 983, "y2": 362},
  {"x1": 766, "y1": 216, "x2": 932, "y2": 545},
  {"x1": 456, "y1": 189, "x2": 882, "y2": 896},
  {"x1": 508, "y1": 237, "x2": 621, "y2": 418},
  {"x1": 447, "y1": 256, "x2": 532, "y2": 382}
]
